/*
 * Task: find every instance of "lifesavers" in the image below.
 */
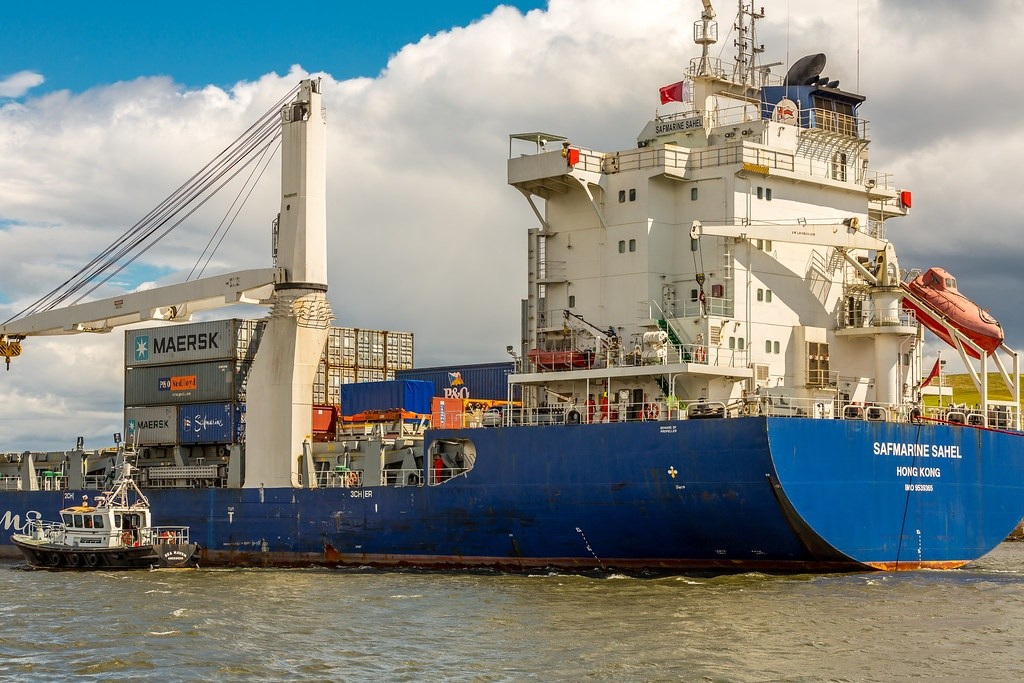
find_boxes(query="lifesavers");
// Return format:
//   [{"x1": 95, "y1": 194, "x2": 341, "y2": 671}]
[
  {"x1": 696, "y1": 349, "x2": 706, "y2": 360},
  {"x1": 348, "y1": 474, "x2": 357, "y2": 486},
  {"x1": 122, "y1": 531, "x2": 140, "y2": 547},
  {"x1": 567, "y1": 411, "x2": 583, "y2": 422},
  {"x1": 163, "y1": 530, "x2": 177, "y2": 544},
  {"x1": 647, "y1": 404, "x2": 658, "y2": 418}
]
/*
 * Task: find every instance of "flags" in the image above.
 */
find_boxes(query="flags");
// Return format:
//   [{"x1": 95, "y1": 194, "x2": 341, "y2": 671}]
[
  {"x1": 659, "y1": 81, "x2": 690, "y2": 105},
  {"x1": 921, "y1": 358, "x2": 939, "y2": 388}
]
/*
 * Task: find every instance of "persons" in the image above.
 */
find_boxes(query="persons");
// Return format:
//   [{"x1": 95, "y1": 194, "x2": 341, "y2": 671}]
[
  {"x1": 585, "y1": 394, "x2": 597, "y2": 423},
  {"x1": 639, "y1": 404, "x2": 649, "y2": 420},
  {"x1": 599, "y1": 392, "x2": 608, "y2": 424},
  {"x1": 163, "y1": 532, "x2": 181, "y2": 545},
  {"x1": 123, "y1": 518, "x2": 133, "y2": 529},
  {"x1": 79, "y1": 515, "x2": 89, "y2": 528},
  {"x1": 433, "y1": 455, "x2": 442, "y2": 483}
]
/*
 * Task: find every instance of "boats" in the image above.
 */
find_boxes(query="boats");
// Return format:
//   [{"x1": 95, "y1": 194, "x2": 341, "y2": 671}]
[
  {"x1": 0, "y1": 0, "x2": 1024, "y2": 575},
  {"x1": 11, "y1": 428, "x2": 202, "y2": 571},
  {"x1": 901, "y1": 267, "x2": 1005, "y2": 361}
]
